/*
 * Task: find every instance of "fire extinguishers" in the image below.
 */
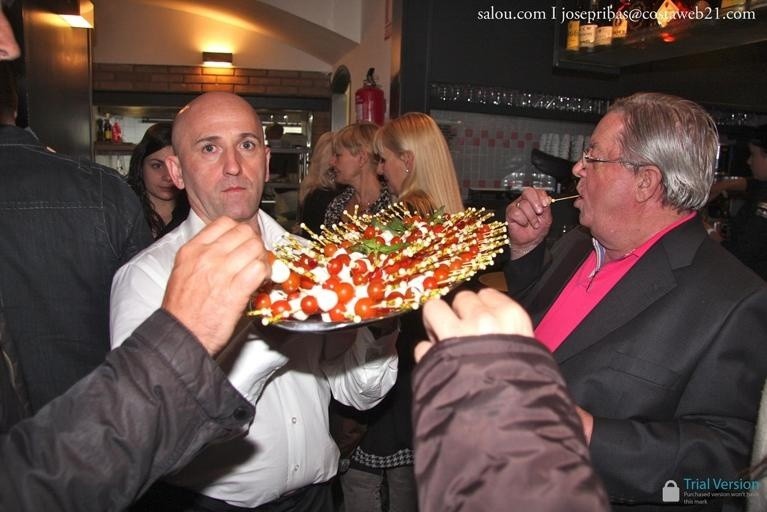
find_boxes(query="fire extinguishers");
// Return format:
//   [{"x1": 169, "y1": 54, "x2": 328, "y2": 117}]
[{"x1": 355, "y1": 66, "x2": 386, "y2": 129}]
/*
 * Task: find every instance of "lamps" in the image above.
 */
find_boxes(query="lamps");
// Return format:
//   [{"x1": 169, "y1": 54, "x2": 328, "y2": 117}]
[
  {"x1": 202, "y1": 52, "x2": 233, "y2": 67},
  {"x1": 58, "y1": 0, "x2": 94, "y2": 29}
]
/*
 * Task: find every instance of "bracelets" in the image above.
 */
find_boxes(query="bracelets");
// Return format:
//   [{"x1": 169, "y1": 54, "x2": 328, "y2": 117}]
[{"x1": 705, "y1": 227, "x2": 716, "y2": 236}]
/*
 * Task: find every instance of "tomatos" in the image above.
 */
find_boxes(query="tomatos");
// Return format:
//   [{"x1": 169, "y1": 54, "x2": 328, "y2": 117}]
[{"x1": 252, "y1": 215, "x2": 490, "y2": 323}]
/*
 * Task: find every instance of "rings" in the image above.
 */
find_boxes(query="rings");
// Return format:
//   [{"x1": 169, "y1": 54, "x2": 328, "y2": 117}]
[{"x1": 515, "y1": 199, "x2": 524, "y2": 211}]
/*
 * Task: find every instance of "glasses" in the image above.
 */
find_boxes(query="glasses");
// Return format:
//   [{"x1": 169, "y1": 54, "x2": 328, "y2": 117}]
[{"x1": 581, "y1": 151, "x2": 625, "y2": 169}]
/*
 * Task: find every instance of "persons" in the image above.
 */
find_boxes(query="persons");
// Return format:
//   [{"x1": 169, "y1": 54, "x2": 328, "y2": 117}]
[
  {"x1": 296, "y1": 131, "x2": 347, "y2": 242},
  {"x1": 125, "y1": 119, "x2": 191, "y2": 240},
  {"x1": 323, "y1": 119, "x2": 401, "y2": 234},
  {"x1": 0, "y1": 1, "x2": 273, "y2": 512},
  {"x1": 339, "y1": 110, "x2": 468, "y2": 512},
  {"x1": 502, "y1": 86, "x2": 765, "y2": 512},
  {"x1": 106, "y1": 88, "x2": 404, "y2": 512},
  {"x1": 0, "y1": 61, "x2": 158, "y2": 443},
  {"x1": 397, "y1": 284, "x2": 616, "y2": 512},
  {"x1": 695, "y1": 123, "x2": 765, "y2": 279}
]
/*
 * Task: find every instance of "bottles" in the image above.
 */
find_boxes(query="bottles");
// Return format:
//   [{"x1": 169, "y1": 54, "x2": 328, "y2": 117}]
[
  {"x1": 103, "y1": 114, "x2": 112, "y2": 141},
  {"x1": 562, "y1": 0, "x2": 716, "y2": 53},
  {"x1": 96, "y1": 119, "x2": 103, "y2": 141},
  {"x1": 111, "y1": 121, "x2": 121, "y2": 142}
]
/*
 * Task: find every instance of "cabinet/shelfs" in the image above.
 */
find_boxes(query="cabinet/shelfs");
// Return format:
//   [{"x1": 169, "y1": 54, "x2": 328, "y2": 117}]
[{"x1": 101, "y1": 108, "x2": 312, "y2": 235}]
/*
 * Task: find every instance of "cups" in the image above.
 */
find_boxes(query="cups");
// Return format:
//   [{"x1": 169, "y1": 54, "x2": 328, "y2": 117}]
[
  {"x1": 434, "y1": 81, "x2": 765, "y2": 129},
  {"x1": 537, "y1": 133, "x2": 592, "y2": 164}
]
